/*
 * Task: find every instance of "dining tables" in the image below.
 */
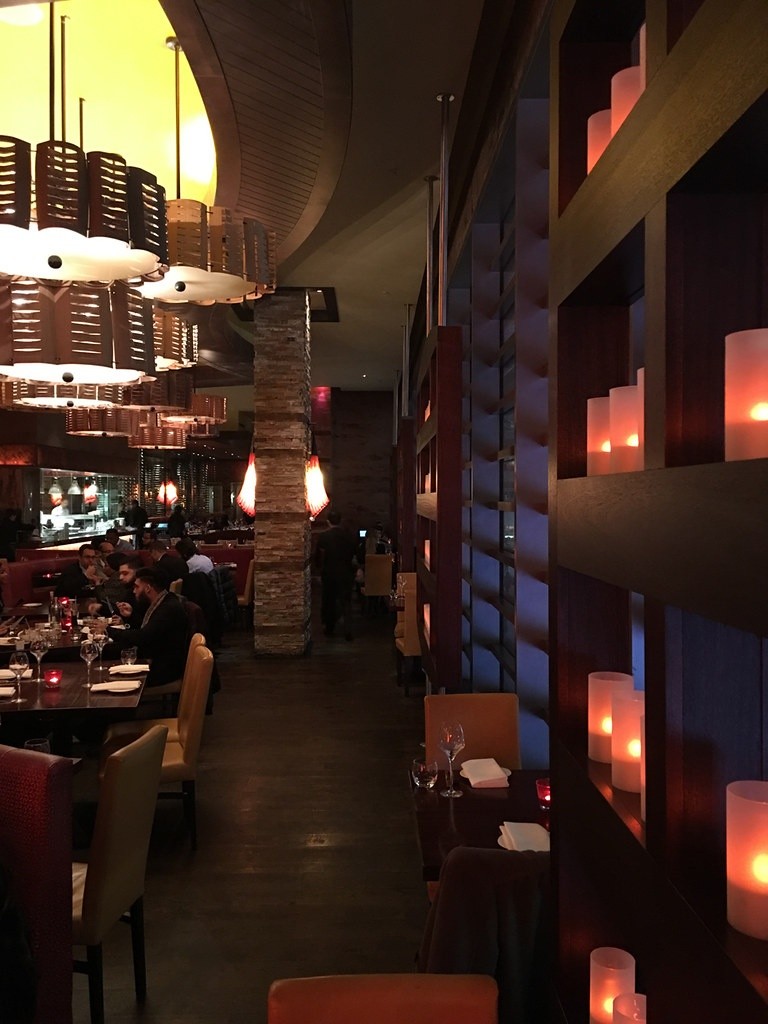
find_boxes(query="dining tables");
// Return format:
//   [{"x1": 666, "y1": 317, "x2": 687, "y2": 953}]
[
  {"x1": 0, "y1": 603, "x2": 89, "y2": 620},
  {"x1": 408, "y1": 770, "x2": 552, "y2": 881},
  {"x1": 2, "y1": 659, "x2": 152, "y2": 718}
]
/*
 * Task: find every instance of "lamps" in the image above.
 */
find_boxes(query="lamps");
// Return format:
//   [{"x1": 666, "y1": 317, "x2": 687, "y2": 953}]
[
  {"x1": 724, "y1": 328, "x2": 768, "y2": 462},
  {"x1": 588, "y1": 947, "x2": 647, "y2": 1024},
  {"x1": 235, "y1": 451, "x2": 258, "y2": 517},
  {"x1": 67, "y1": 478, "x2": 82, "y2": 495},
  {"x1": 586, "y1": 65, "x2": 642, "y2": 175},
  {"x1": 304, "y1": 428, "x2": 331, "y2": 521},
  {"x1": 585, "y1": 385, "x2": 645, "y2": 477},
  {"x1": 47, "y1": 480, "x2": 62, "y2": 494},
  {"x1": 587, "y1": 672, "x2": 645, "y2": 793},
  {"x1": 726, "y1": 780, "x2": 768, "y2": 941},
  {"x1": 0, "y1": 0, "x2": 277, "y2": 449}
]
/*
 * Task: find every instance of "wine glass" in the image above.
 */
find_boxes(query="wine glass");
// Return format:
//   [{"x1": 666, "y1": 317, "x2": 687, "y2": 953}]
[
  {"x1": 9, "y1": 652, "x2": 29, "y2": 703},
  {"x1": 93, "y1": 628, "x2": 109, "y2": 670},
  {"x1": 80, "y1": 643, "x2": 98, "y2": 688},
  {"x1": 439, "y1": 722, "x2": 465, "y2": 797},
  {"x1": 30, "y1": 635, "x2": 48, "y2": 682}
]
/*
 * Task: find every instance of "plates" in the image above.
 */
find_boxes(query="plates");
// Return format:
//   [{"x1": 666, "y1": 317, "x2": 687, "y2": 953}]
[
  {"x1": 23, "y1": 602, "x2": 42, "y2": 606},
  {"x1": 108, "y1": 689, "x2": 136, "y2": 692},
  {"x1": 118, "y1": 670, "x2": 142, "y2": 674},
  {"x1": 459, "y1": 768, "x2": 510, "y2": 778},
  {"x1": 0, "y1": 637, "x2": 29, "y2": 645}
]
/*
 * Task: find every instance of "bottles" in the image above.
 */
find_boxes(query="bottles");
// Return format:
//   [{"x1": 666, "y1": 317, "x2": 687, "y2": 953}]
[{"x1": 47, "y1": 591, "x2": 60, "y2": 622}]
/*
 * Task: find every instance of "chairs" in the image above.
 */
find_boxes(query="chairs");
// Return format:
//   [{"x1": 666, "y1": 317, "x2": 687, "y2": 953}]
[
  {"x1": 72, "y1": 724, "x2": 169, "y2": 1024},
  {"x1": 423, "y1": 693, "x2": 521, "y2": 771},
  {"x1": 396, "y1": 572, "x2": 423, "y2": 698},
  {"x1": 361, "y1": 554, "x2": 392, "y2": 618},
  {"x1": 73, "y1": 633, "x2": 213, "y2": 842},
  {"x1": 418, "y1": 846, "x2": 551, "y2": 1024},
  {"x1": 0, "y1": 744, "x2": 72, "y2": 1024},
  {"x1": 267, "y1": 973, "x2": 498, "y2": 1024}
]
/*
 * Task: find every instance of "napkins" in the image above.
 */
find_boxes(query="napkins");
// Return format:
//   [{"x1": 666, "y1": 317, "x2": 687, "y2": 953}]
[
  {"x1": 459, "y1": 759, "x2": 511, "y2": 791},
  {"x1": 497, "y1": 820, "x2": 551, "y2": 853},
  {"x1": 108, "y1": 665, "x2": 150, "y2": 674},
  {"x1": 0, "y1": 687, "x2": 16, "y2": 697},
  {"x1": 0, "y1": 668, "x2": 33, "y2": 679},
  {"x1": 90, "y1": 681, "x2": 141, "y2": 690}
]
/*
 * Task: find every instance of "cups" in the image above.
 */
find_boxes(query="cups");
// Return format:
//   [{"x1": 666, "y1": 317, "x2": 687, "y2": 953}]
[
  {"x1": 389, "y1": 589, "x2": 398, "y2": 599},
  {"x1": 44, "y1": 668, "x2": 62, "y2": 688},
  {"x1": 82, "y1": 597, "x2": 107, "y2": 634},
  {"x1": 34, "y1": 621, "x2": 61, "y2": 647},
  {"x1": 411, "y1": 757, "x2": 438, "y2": 789},
  {"x1": 536, "y1": 779, "x2": 551, "y2": 810},
  {"x1": 193, "y1": 540, "x2": 205, "y2": 550},
  {"x1": 217, "y1": 540, "x2": 237, "y2": 549},
  {"x1": 120, "y1": 647, "x2": 137, "y2": 665}
]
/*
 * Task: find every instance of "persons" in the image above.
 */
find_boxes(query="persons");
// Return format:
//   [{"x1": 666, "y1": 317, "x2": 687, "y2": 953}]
[
  {"x1": 167, "y1": 505, "x2": 187, "y2": 539},
  {"x1": 315, "y1": 512, "x2": 355, "y2": 642},
  {"x1": 149, "y1": 540, "x2": 189, "y2": 576},
  {"x1": 141, "y1": 532, "x2": 153, "y2": 550},
  {"x1": 51, "y1": 498, "x2": 69, "y2": 515},
  {"x1": 0, "y1": 562, "x2": 8, "y2": 636},
  {"x1": 357, "y1": 530, "x2": 396, "y2": 616},
  {"x1": 125, "y1": 500, "x2": 148, "y2": 541},
  {"x1": 206, "y1": 512, "x2": 254, "y2": 530},
  {"x1": 58, "y1": 528, "x2": 151, "y2": 631},
  {"x1": 105, "y1": 566, "x2": 190, "y2": 688},
  {"x1": 0, "y1": 508, "x2": 36, "y2": 562},
  {"x1": 175, "y1": 537, "x2": 214, "y2": 573}
]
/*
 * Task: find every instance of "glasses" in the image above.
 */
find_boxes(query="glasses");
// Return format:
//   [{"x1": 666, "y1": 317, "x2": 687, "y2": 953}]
[{"x1": 81, "y1": 555, "x2": 96, "y2": 560}]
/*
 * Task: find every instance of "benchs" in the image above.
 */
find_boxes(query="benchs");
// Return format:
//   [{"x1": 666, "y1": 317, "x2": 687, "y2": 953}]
[{"x1": 1, "y1": 548, "x2": 255, "y2": 632}]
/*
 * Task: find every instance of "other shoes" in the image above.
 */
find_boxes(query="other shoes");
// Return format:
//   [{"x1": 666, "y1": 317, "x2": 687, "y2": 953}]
[
  {"x1": 345, "y1": 631, "x2": 354, "y2": 641},
  {"x1": 323, "y1": 625, "x2": 334, "y2": 636}
]
